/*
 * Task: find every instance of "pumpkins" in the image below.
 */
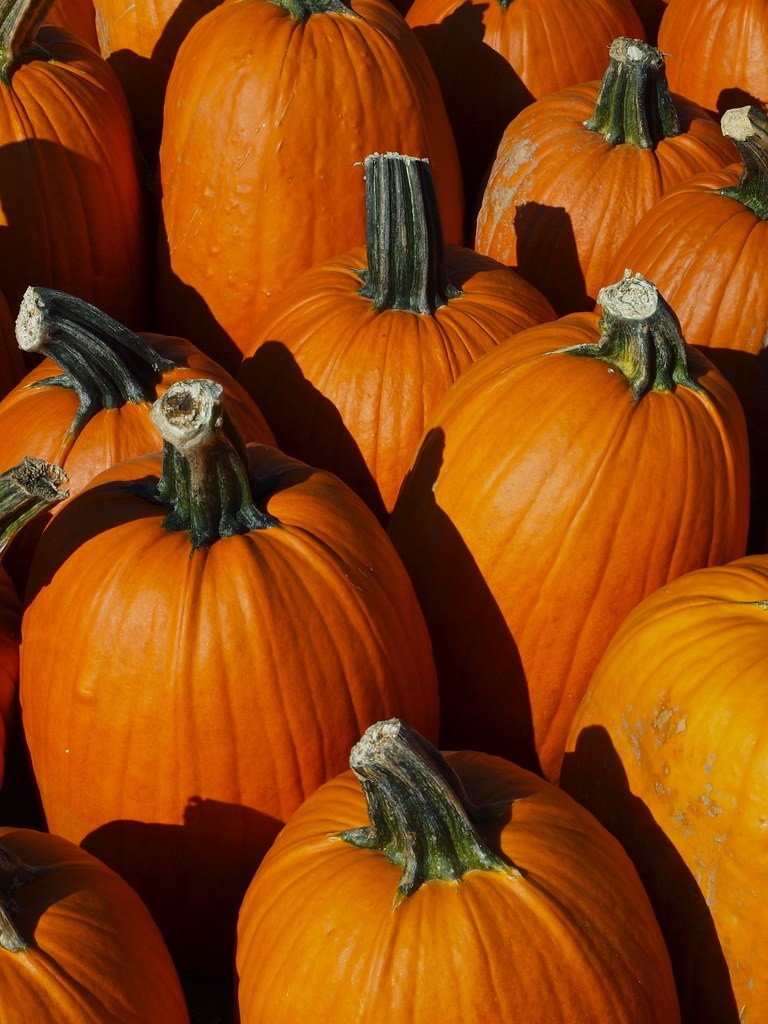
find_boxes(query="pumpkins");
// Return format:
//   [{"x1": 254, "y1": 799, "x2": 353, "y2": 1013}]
[{"x1": 0, "y1": 0, "x2": 768, "y2": 1024}]
